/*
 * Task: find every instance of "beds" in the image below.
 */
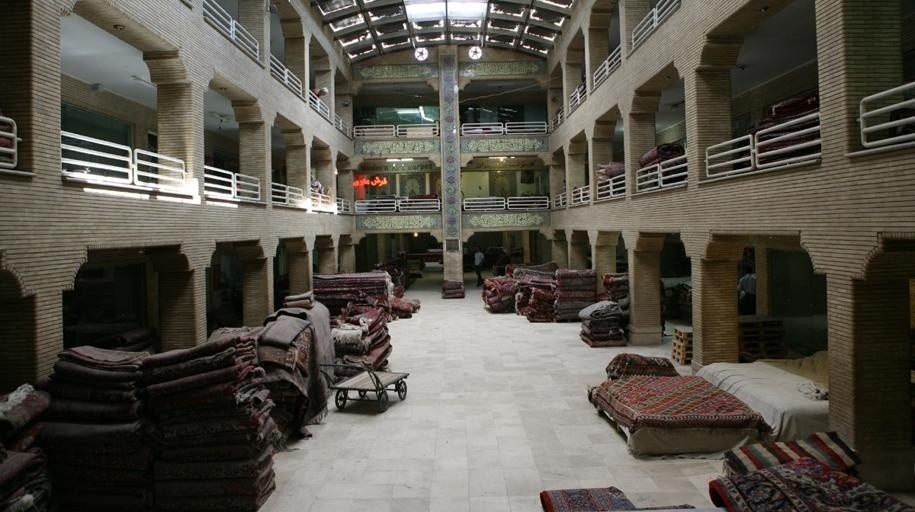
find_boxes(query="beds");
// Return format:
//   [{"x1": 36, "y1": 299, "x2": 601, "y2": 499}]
[
  {"x1": 584, "y1": 374, "x2": 771, "y2": 458},
  {"x1": 697, "y1": 344, "x2": 834, "y2": 447}
]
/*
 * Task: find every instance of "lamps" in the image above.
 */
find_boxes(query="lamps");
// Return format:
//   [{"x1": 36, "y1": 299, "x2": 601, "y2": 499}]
[
  {"x1": 413, "y1": 46, "x2": 429, "y2": 61},
  {"x1": 468, "y1": 45, "x2": 483, "y2": 60}
]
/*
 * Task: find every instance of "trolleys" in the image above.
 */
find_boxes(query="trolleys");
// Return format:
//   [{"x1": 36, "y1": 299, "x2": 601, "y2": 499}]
[{"x1": 318, "y1": 361, "x2": 410, "y2": 413}]
